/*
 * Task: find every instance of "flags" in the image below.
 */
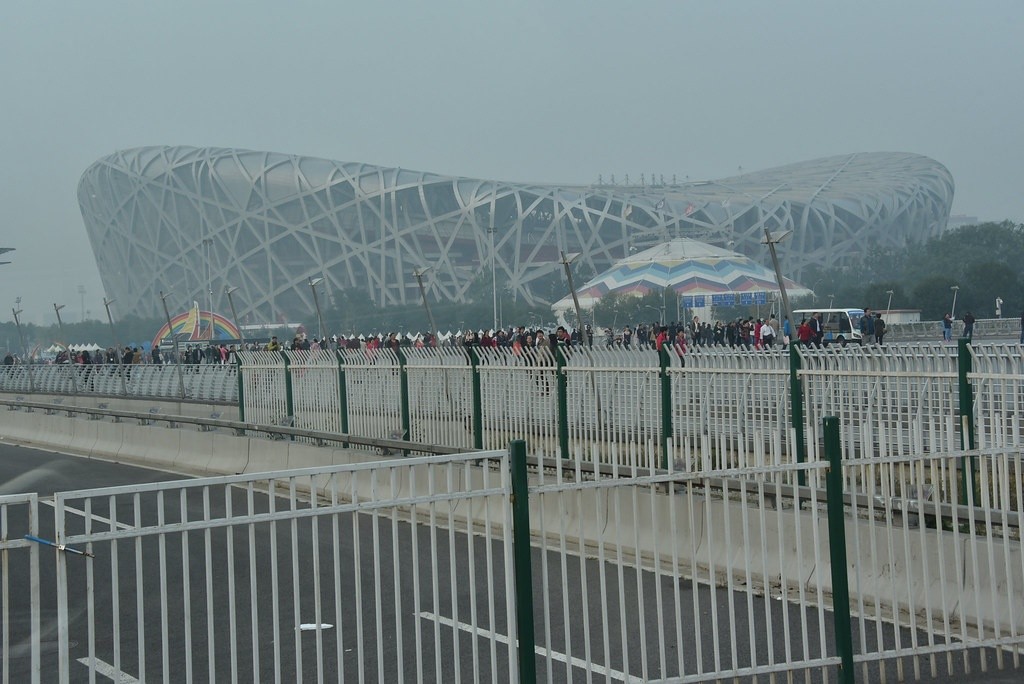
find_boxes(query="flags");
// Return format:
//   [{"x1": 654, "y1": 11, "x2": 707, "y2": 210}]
[
  {"x1": 655, "y1": 197, "x2": 665, "y2": 210},
  {"x1": 686, "y1": 201, "x2": 694, "y2": 215}
]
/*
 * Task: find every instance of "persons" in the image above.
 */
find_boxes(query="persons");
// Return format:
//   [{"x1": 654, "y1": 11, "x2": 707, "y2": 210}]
[
  {"x1": 655, "y1": 327, "x2": 671, "y2": 377},
  {"x1": 152, "y1": 345, "x2": 164, "y2": 371},
  {"x1": 164, "y1": 323, "x2": 594, "y2": 393},
  {"x1": 963, "y1": 312, "x2": 975, "y2": 339},
  {"x1": 622, "y1": 325, "x2": 633, "y2": 351},
  {"x1": 4, "y1": 346, "x2": 153, "y2": 391},
  {"x1": 941, "y1": 313, "x2": 955, "y2": 343},
  {"x1": 605, "y1": 328, "x2": 622, "y2": 350},
  {"x1": 1020, "y1": 312, "x2": 1024, "y2": 349},
  {"x1": 633, "y1": 308, "x2": 885, "y2": 351},
  {"x1": 673, "y1": 326, "x2": 688, "y2": 378},
  {"x1": 689, "y1": 315, "x2": 702, "y2": 359}
]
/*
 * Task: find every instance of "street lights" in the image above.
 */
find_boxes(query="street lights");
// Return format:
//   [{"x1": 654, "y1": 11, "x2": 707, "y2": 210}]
[
  {"x1": 767, "y1": 298, "x2": 775, "y2": 324},
  {"x1": 53, "y1": 303, "x2": 73, "y2": 364},
  {"x1": 307, "y1": 276, "x2": 331, "y2": 350},
  {"x1": 558, "y1": 249, "x2": 590, "y2": 348},
  {"x1": 826, "y1": 294, "x2": 836, "y2": 324},
  {"x1": 947, "y1": 286, "x2": 961, "y2": 318},
  {"x1": 886, "y1": 289, "x2": 895, "y2": 323},
  {"x1": 643, "y1": 304, "x2": 663, "y2": 326},
  {"x1": 528, "y1": 312, "x2": 543, "y2": 328},
  {"x1": 612, "y1": 310, "x2": 620, "y2": 332},
  {"x1": 759, "y1": 226, "x2": 798, "y2": 341},
  {"x1": 102, "y1": 296, "x2": 123, "y2": 365},
  {"x1": 159, "y1": 291, "x2": 180, "y2": 365},
  {"x1": 223, "y1": 286, "x2": 247, "y2": 350},
  {"x1": 411, "y1": 266, "x2": 442, "y2": 347},
  {"x1": 710, "y1": 308, "x2": 716, "y2": 327},
  {"x1": 11, "y1": 308, "x2": 29, "y2": 364}
]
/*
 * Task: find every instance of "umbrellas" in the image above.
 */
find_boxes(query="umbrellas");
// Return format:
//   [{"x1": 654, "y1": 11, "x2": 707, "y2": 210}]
[
  {"x1": 45, "y1": 343, "x2": 107, "y2": 352},
  {"x1": 551, "y1": 235, "x2": 814, "y2": 310}
]
